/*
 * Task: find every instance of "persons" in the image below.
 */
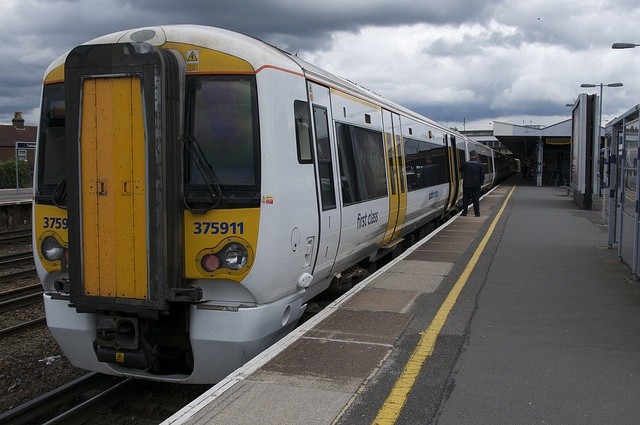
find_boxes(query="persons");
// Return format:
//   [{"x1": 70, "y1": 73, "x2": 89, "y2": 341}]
[
  {"x1": 521, "y1": 164, "x2": 528, "y2": 180},
  {"x1": 459, "y1": 149, "x2": 485, "y2": 218}
]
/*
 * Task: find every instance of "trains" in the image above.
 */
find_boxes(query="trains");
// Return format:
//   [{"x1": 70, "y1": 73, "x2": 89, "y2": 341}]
[{"x1": 32, "y1": 25, "x2": 516, "y2": 385}]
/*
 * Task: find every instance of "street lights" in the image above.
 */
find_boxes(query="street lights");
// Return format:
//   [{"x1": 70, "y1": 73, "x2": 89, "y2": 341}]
[
  {"x1": 612, "y1": 42, "x2": 640, "y2": 48},
  {"x1": 581, "y1": 83, "x2": 623, "y2": 195}
]
